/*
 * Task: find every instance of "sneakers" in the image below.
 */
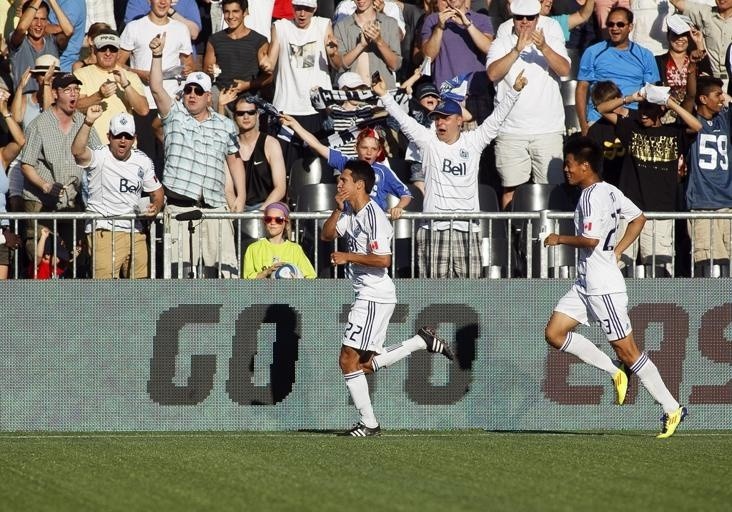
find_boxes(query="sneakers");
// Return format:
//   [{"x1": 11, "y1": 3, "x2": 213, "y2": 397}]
[
  {"x1": 343, "y1": 421, "x2": 381, "y2": 437},
  {"x1": 612, "y1": 362, "x2": 630, "y2": 405},
  {"x1": 418, "y1": 326, "x2": 455, "y2": 361},
  {"x1": 656, "y1": 404, "x2": 688, "y2": 439}
]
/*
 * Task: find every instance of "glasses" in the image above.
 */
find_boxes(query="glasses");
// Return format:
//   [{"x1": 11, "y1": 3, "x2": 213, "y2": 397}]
[
  {"x1": 514, "y1": 14, "x2": 536, "y2": 21},
  {"x1": 112, "y1": 133, "x2": 133, "y2": 140},
  {"x1": 57, "y1": 87, "x2": 81, "y2": 94},
  {"x1": 99, "y1": 45, "x2": 119, "y2": 53},
  {"x1": 183, "y1": 86, "x2": 204, "y2": 96},
  {"x1": 265, "y1": 216, "x2": 287, "y2": 224},
  {"x1": 234, "y1": 109, "x2": 256, "y2": 116},
  {"x1": 606, "y1": 21, "x2": 631, "y2": 27}
]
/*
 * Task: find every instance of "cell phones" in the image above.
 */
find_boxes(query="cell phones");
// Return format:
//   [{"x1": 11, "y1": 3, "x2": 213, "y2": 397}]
[{"x1": 372, "y1": 71, "x2": 380, "y2": 84}]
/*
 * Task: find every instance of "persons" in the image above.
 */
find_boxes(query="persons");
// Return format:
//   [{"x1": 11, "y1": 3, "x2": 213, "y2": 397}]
[
  {"x1": 315, "y1": 155, "x2": 458, "y2": 440},
  {"x1": 543, "y1": 132, "x2": 690, "y2": 442},
  {"x1": 242, "y1": 198, "x2": 316, "y2": 280},
  {"x1": 1, "y1": 1, "x2": 732, "y2": 278}
]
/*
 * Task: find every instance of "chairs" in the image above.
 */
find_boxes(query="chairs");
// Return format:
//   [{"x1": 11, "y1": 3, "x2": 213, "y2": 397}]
[
  {"x1": 385, "y1": 182, "x2": 578, "y2": 278},
  {"x1": 288, "y1": 157, "x2": 347, "y2": 277}
]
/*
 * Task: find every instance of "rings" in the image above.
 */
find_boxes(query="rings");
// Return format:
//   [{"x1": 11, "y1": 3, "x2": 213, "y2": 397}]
[{"x1": 330, "y1": 258, "x2": 335, "y2": 263}]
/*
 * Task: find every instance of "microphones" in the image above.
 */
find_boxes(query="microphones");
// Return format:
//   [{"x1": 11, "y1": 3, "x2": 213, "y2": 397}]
[{"x1": 175, "y1": 209, "x2": 203, "y2": 221}]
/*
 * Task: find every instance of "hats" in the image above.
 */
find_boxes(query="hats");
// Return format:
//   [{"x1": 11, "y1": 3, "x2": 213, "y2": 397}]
[
  {"x1": 338, "y1": 71, "x2": 369, "y2": 90},
  {"x1": 356, "y1": 127, "x2": 385, "y2": 144},
  {"x1": 51, "y1": 71, "x2": 82, "y2": 89},
  {"x1": 426, "y1": 100, "x2": 462, "y2": 118},
  {"x1": 416, "y1": 83, "x2": 440, "y2": 101},
  {"x1": 29, "y1": 53, "x2": 61, "y2": 73},
  {"x1": 292, "y1": 0, "x2": 318, "y2": 8},
  {"x1": 93, "y1": 33, "x2": 120, "y2": 48},
  {"x1": 109, "y1": 111, "x2": 136, "y2": 137},
  {"x1": 184, "y1": 71, "x2": 212, "y2": 92},
  {"x1": 510, "y1": 0, "x2": 542, "y2": 17}
]
